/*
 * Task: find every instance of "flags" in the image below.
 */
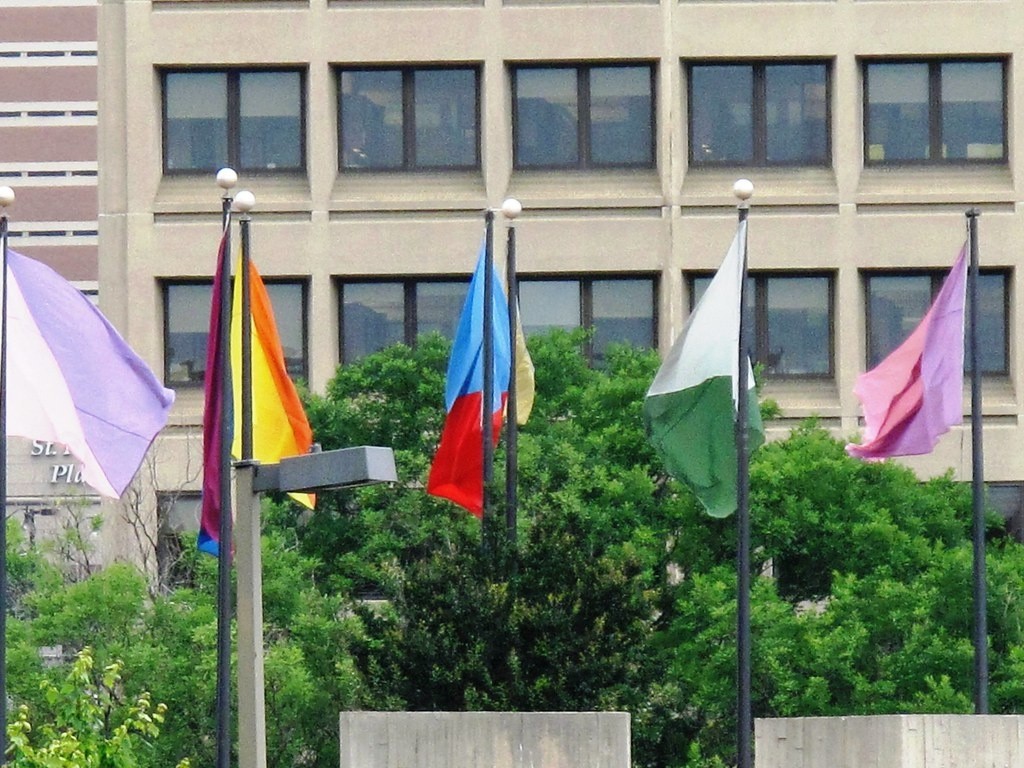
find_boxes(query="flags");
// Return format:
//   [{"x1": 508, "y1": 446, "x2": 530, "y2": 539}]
[
  {"x1": 195, "y1": 237, "x2": 318, "y2": 553},
  {"x1": 645, "y1": 221, "x2": 766, "y2": 517},
  {"x1": 846, "y1": 243, "x2": 969, "y2": 459},
  {"x1": 427, "y1": 244, "x2": 511, "y2": 519},
  {"x1": 4, "y1": 249, "x2": 176, "y2": 501},
  {"x1": 504, "y1": 262, "x2": 534, "y2": 425}
]
